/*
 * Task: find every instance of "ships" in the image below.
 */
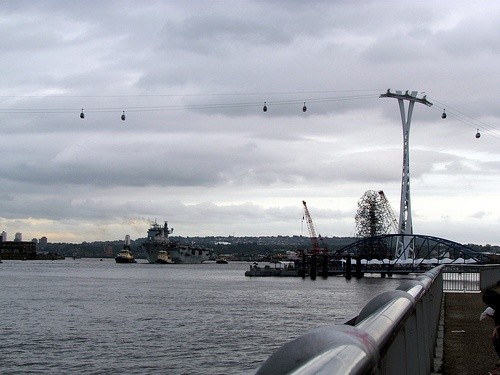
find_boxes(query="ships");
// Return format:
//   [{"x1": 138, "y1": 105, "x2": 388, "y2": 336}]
[{"x1": 139, "y1": 218, "x2": 214, "y2": 264}]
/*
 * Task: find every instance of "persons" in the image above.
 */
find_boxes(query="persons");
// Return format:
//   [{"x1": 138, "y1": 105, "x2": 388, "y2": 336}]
[
  {"x1": 253, "y1": 262, "x2": 294, "y2": 271},
  {"x1": 481, "y1": 279, "x2": 500, "y2": 346}
]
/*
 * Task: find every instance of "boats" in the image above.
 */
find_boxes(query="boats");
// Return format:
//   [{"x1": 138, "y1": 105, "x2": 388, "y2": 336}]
[
  {"x1": 245, "y1": 257, "x2": 319, "y2": 277},
  {"x1": 216, "y1": 257, "x2": 228, "y2": 264},
  {"x1": 114, "y1": 245, "x2": 137, "y2": 263},
  {"x1": 156, "y1": 250, "x2": 177, "y2": 264}
]
